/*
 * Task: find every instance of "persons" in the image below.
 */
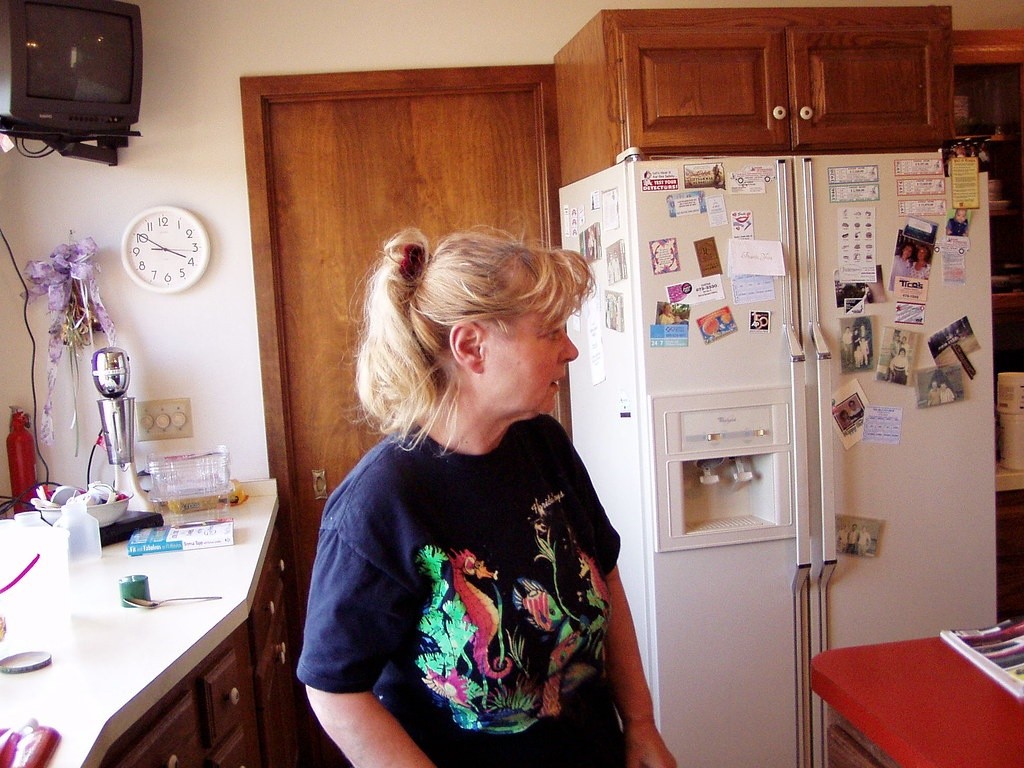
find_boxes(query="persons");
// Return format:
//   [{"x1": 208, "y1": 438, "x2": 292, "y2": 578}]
[
  {"x1": 656, "y1": 135, "x2": 994, "y2": 557},
  {"x1": 295, "y1": 225, "x2": 679, "y2": 768}
]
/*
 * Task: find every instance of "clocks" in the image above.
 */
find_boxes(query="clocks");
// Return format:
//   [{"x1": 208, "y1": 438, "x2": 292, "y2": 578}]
[{"x1": 120, "y1": 206, "x2": 211, "y2": 294}]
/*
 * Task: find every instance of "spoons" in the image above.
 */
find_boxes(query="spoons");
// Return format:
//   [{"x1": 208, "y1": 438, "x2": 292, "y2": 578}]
[{"x1": 119, "y1": 594, "x2": 224, "y2": 609}]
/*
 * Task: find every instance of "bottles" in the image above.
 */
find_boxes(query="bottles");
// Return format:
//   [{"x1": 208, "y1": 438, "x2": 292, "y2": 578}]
[{"x1": 53, "y1": 505, "x2": 101, "y2": 558}]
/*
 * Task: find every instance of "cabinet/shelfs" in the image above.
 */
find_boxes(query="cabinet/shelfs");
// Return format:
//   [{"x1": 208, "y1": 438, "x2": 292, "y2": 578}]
[
  {"x1": 80, "y1": 480, "x2": 300, "y2": 768},
  {"x1": 811, "y1": 636, "x2": 1024, "y2": 768},
  {"x1": 554, "y1": 6, "x2": 956, "y2": 186},
  {"x1": 948, "y1": 27, "x2": 1024, "y2": 626}
]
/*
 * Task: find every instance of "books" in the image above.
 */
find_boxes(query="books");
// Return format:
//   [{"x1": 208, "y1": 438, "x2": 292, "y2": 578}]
[
  {"x1": 127, "y1": 517, "x2": 234, "y2": 556},
  {"x1": 939, "y1": 619, "x2": 1024, "y2": 698}
]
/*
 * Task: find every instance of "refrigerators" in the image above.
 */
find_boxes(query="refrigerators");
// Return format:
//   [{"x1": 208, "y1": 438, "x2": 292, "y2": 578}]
[{"x1": 559, "y1": 158, "x2": 999, "y2": 768}]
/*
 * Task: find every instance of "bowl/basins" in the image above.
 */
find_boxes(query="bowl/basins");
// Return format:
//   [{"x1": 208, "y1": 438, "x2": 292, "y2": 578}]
[{"x1": 36, "y1": 490, "x2": 134, "y2": 526}]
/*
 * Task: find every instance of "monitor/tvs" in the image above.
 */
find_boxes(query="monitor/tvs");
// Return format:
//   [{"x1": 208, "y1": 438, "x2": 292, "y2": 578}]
[{"x1": 0, "y1": 0, "x2": 144, "y2": 135}]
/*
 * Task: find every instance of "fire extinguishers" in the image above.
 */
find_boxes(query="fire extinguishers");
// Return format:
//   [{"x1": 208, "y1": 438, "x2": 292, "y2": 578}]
[{"x1": 6, "y1": 405, "x2": 39, "y2": 515}]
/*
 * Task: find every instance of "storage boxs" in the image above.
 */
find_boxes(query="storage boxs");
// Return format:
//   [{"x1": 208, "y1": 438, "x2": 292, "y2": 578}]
[{"x1": 127, "y1": 518, "x2": 234, "y2": 556}]
